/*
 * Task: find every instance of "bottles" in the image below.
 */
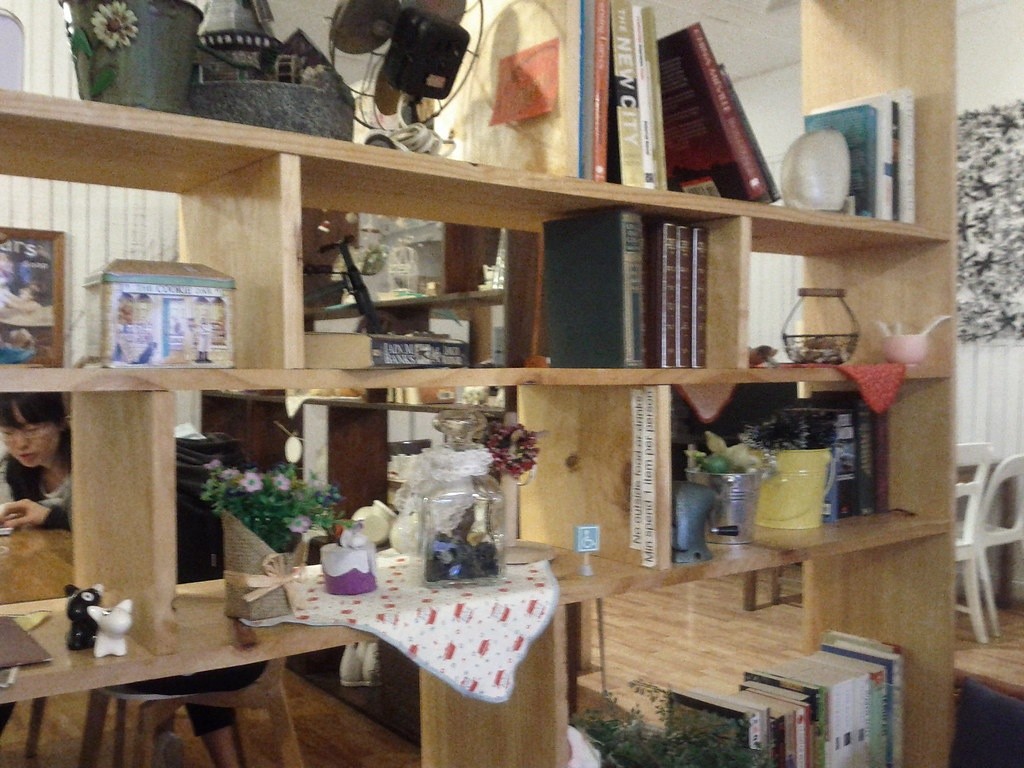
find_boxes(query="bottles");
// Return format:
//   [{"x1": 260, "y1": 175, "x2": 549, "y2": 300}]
[
  {"x1": 352, "y1": 499, "x2": 397, "y2": 548},
  {"x1": 340, "y1": 640, "x2": 381, "y2": 687},
  {"x1": 780, "y1": 287, "x2": 860, "y2": 364},
  {"x1": 422, "y1": 411, "x2": 506, "y2": 588}
]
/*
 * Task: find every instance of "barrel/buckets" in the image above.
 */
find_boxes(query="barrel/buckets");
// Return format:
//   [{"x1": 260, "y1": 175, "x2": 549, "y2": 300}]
[
  {"x1": 740, "y1": 446, "x2": 836, "y2": 529},
  {"x1": 685, "y1": 469, "x2": 766, "y2": 543},
  {"x1": 58, "y1": 1, "x2": 204, "y2": 114}
]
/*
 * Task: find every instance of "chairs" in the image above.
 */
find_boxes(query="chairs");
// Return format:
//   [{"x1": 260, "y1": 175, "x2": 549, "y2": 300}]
[
  {"x1": 957, "y1": 453, "x2": 1024, "y2": 637},
  {"x1": 954, "y1": 441, "x2": 994, "y2": 643},
  {"x1": 84, "y1": 654, "x2": 307, "y2": 768}
]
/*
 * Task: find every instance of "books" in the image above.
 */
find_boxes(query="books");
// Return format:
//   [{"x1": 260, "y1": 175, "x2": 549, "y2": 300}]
[
  {"x1": 769, "y1": 394, "x2": 890, "y2": 525},
  {"x1": 540, "y1": 211, "x2": 710, "y2": 369},
  {"x1": 591, "y1": 0, "x2": 781, "y2": 205},
  {"x1": 804, "y1": 89, "x2": 918, "y2": 223},
  {"x1": 302, "y1": 331, "x2": 467, "y2": 372},
  {"x1": 667, "y1": 629, "x2": 905, "y2": 768}
]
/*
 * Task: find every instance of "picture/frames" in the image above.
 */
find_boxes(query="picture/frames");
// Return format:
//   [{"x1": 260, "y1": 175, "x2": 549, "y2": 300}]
[{"x1": 0, "y1": 226, "x2": 66, "y2": 372}]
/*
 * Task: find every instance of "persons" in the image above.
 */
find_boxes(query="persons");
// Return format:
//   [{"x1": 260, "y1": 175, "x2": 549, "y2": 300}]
[{"x1": 0, "y1": 390, "x2": 281, "y2": 768}]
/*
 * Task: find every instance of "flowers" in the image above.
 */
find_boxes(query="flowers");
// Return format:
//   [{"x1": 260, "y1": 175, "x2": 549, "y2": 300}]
[
  {"x1": 472, "y1": 420, "x2": 542, "y2": 485},
  {"x1": 203, "y1": 454, "x2": 364, "y2": 550},
  {"x1": 67, "y1": 0, "x2": 138, "y2": 102}
]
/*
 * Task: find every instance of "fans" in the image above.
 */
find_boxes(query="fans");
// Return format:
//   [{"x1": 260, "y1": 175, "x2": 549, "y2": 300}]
[{"x1": 329, "y1": 0, "x2": 487, "y2": 129}]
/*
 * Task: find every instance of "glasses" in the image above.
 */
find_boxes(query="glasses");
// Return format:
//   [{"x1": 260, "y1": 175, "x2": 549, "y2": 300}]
[{"x1": 0, "y1": 424, "x2": 46, "y2": 442}]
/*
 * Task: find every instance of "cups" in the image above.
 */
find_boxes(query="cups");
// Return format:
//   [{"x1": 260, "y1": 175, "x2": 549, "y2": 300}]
[{"x1": 881, "y1": 334, "x2": 930, "y2": 367}]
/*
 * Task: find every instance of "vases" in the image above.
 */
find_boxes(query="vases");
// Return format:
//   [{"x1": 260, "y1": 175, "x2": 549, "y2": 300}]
[
  {"x1": 410, "y1": 411, "x2": 506, "y2": 585},
  {"x1": 219, "y1": 511, "x2": 307, "y2": 620}
]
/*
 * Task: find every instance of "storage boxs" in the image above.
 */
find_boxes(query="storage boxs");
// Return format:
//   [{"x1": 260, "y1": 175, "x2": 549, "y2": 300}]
[{"x1": 82, "y1": 257, "x2": 239, "y2": 374}]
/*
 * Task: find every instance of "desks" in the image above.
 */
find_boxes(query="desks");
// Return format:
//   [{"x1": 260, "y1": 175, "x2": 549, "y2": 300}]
[{"x1": 0, "y1": 525, "x2": 75, "y2": 606}]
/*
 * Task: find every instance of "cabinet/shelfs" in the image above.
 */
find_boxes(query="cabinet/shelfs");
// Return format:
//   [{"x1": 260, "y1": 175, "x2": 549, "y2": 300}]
[{"x1": 0, "y1": 0, "x2": 957, "y2": 768}]
